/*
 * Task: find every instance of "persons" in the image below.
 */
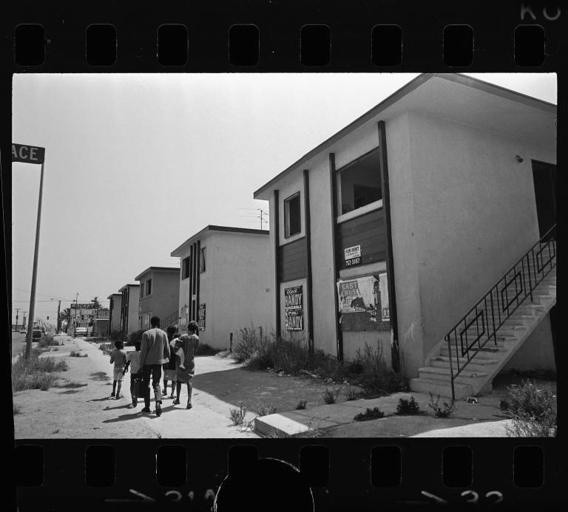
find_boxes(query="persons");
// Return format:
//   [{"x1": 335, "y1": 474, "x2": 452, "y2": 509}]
[
  {"x1": 110, "y1": 341, "x2": 128, "y2": 399},
  {"x1": 123, "y1": 316, "x2": 200, "y2": 416}
]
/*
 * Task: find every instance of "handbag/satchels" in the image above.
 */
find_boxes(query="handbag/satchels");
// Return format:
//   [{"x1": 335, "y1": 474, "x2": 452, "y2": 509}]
[{"x1": 133, "y1": 369, "x2": 146, "y2": 397}]
